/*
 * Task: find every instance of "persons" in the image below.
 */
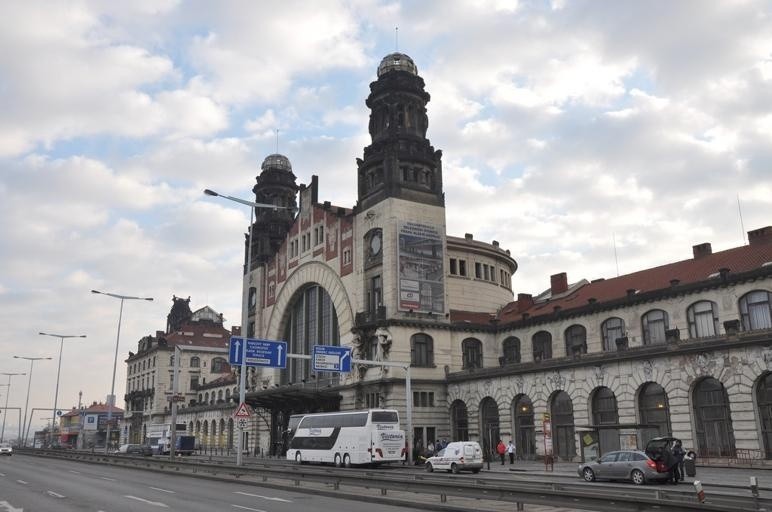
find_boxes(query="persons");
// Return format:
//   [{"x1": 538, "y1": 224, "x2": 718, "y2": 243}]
[
  {"x1": 497, "y1": 441, "x2": 516, "y2": 465},
  {"x1": 416, "y1": 438, "x2": 447, "y2": 456},
  {"x1": 657, "y1": 439, "x2": 686, "y2": 484}
]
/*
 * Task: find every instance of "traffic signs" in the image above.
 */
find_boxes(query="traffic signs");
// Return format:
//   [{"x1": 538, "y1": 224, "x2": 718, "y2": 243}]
[
  {"x1": 229, "y1": 336, "x2": 288, "y2": 369},
  {"x1": 313, "y1": 345, "x2": 351, "y2": 372}
]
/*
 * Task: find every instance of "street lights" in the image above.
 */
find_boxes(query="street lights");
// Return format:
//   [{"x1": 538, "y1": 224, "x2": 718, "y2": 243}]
[
  {"x1": 0, "y1": 373, "x2": 28, "y2": 446},
  {"x1": 38, "y1": 332, "x2": 87, "y2": 444},
  {"x1": 203, "y1": 188, "x2": 300, "y2": 466},
  {"x1": 13, "y1": 356, "x2": 54, "y2": 447},
  {"x1": 91, "y1": 289, "x2": 154, "y2": 455}
]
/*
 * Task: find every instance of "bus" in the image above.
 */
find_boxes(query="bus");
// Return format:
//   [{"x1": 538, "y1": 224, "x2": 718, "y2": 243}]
[{"x1": 286, "y1": 409, "x2": 406, "y2": 468}]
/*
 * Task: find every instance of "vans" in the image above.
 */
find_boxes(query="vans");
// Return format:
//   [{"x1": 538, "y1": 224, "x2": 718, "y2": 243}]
[
  {"x1": 158, "y1": 430, "x2": 195, "y2": 456},
  {"x1": 425, "y1": 441, "x2": 484, "y2": 474}
]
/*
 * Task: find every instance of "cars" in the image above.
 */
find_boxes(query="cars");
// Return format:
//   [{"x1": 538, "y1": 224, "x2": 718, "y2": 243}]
[
  {"x1": 577, "y1": 437, "x2": 683, "y2": 484},
  {"x1": 0, "y1": 443, "x2": 13, "y2": 456},
  {"x1": 113, "y1": 442, "x2": 153, "y2": 456}
]
